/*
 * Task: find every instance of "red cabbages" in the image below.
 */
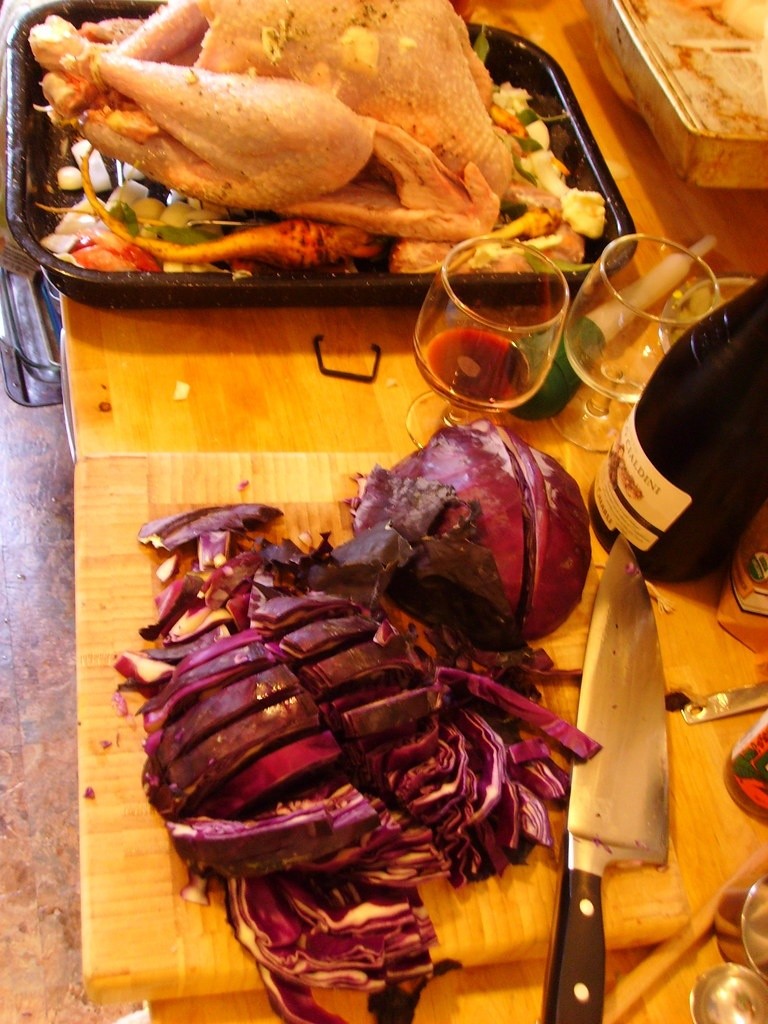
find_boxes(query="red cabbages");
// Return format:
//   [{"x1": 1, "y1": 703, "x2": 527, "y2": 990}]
[{"x1": 120, "y1": 425, "x2": 600, "y2": 1023}]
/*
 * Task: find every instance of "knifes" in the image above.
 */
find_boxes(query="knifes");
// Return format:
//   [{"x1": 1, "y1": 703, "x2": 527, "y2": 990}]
[{"x1": 540, "y1": 535, "x2": 667, "y2": 1023}]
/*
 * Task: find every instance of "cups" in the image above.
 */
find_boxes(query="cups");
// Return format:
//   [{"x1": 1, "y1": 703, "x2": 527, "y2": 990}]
[
  {"x1": 724, "y1": 711, "x2": 768, "y2": 817},
  {"x1": 715, "y1": 868, "x2": 768, "y2": 981},
  {"x1": 659, "y1": 273, "x2": 755, "y2": 353}
]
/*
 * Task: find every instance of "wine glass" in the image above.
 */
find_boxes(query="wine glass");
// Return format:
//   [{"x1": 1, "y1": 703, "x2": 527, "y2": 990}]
[
  {"x1": 405, "y1": 237, "x2": 569, "y2": 448},
  {"x1": 551, "y1": 236, "x2": 718, "y2": 452}
]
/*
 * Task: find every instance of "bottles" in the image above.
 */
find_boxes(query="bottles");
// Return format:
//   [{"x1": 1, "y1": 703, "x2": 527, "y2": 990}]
[{"x1": 590, "y1": 277, "x2": 768, "y2": 582}]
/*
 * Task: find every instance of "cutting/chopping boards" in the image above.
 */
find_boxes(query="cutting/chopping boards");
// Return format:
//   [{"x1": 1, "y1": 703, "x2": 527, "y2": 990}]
[{"x1": 76, "y1": 451, "x2": 768, "y2": 997}]
